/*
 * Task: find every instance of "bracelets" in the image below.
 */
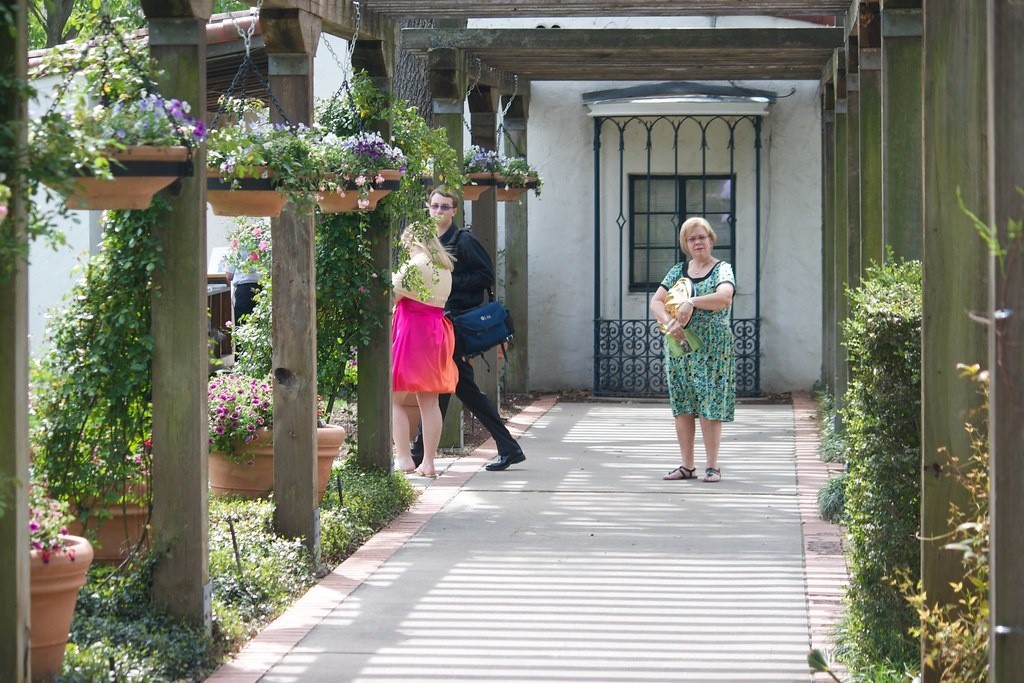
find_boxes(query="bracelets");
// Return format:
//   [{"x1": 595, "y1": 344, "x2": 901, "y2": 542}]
[
  {"x1": 667, "y1": 318, "x2": 676, "y2": 330},
  {"x1": 687, "y1": 299, "x2": 694, "y2": 307}
]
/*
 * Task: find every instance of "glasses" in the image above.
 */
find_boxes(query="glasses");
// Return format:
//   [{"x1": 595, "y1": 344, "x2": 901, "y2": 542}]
[
  {"x1": 430, "y1": 205, "x2": 454, "y2": 210},
  {"x1": 686, "y1": 234, "x2": 711, "y2": 240}
]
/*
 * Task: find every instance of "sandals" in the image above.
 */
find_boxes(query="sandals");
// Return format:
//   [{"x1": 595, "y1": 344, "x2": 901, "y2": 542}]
[
  {"x1": 662, "y1": 466, "x2": 698, "y2": 480},
  {"x1": 701, "y1": 467, "x2": 722, "y2": 482}
]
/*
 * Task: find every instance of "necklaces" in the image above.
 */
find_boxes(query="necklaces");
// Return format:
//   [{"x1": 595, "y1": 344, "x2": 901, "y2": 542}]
[{"x1": 694, "y1": 264, "x2": 704, "y2": 274}]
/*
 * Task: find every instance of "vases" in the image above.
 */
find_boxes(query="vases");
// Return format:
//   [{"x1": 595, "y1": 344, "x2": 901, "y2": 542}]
[
  {"x1": 317, "y1": 168, "x2": 403, "y2": 214},
  {"x1": 207, "y1": 424, "x2": 344, "y2": 508},
  {"x1": 31, "y1": 145, "x2": 193, "y2": 211},
  {"x1": 204, "y1": 162, "x2": 301, "y2": 218},
  {"x1": 459, "y1": 173, "x2": 493, "y2": 201},
  {"x1": 496, "y1": 173, "x2": 535, "y2": 203},
  {"x1": 29, "y1": 533, "x2": 93, "y2": 683},
  {"x1": 61, "y1": 476, "x2": 151, "y2": 565}
]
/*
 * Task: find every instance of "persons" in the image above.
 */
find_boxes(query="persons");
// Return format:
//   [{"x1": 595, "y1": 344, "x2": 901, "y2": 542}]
[
  {"x1": 649, "y1": 216, "x2": 736, "y2": 483},
  {"x1": 390, "y1": 220, "x2": 459, "y2": 476},
  {"x1": 410, "y1": 187, "x2": 525, "y2": 472},
  {"x1": 225, "y1": 249, "x2": 263, "y2": 364}
]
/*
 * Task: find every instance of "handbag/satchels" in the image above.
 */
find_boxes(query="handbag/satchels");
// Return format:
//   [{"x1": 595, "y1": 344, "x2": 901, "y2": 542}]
[
  {"x1": 657, "y1": 277, "x2": 695, "y2": 334},
  {"x1": 452, "y1": 301, "x2": 516, "y2": 373}
]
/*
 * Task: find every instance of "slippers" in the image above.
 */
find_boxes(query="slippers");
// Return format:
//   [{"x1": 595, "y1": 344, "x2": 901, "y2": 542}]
[{"x1": 394, "y1": 466, "x2": 437, "y2": 477}]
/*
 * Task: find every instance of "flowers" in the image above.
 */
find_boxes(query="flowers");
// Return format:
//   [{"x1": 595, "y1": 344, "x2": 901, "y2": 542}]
[
  {"x1": 27, "y1": 424, "x2": 152, "y2": 564},
  {"x1": 207, "y1": 369, "x2": 273, "y2": 468},
  {"x1": 37, "y1": 88, "x2": 546, "y2": 219}
]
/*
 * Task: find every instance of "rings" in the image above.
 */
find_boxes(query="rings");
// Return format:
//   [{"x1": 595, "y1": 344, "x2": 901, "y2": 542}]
[
  {"x1": 674, "y1": 333, "x2": 677, "y2": 336},
  {"x1": 682, "y1": 315, "x2": 685, "y2": 318}
]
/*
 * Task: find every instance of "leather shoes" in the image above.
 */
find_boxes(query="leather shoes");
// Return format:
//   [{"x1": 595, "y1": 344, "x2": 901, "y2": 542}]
[{"x1": 485, "y1": 448, "x2": 526, "y2": 471}]
